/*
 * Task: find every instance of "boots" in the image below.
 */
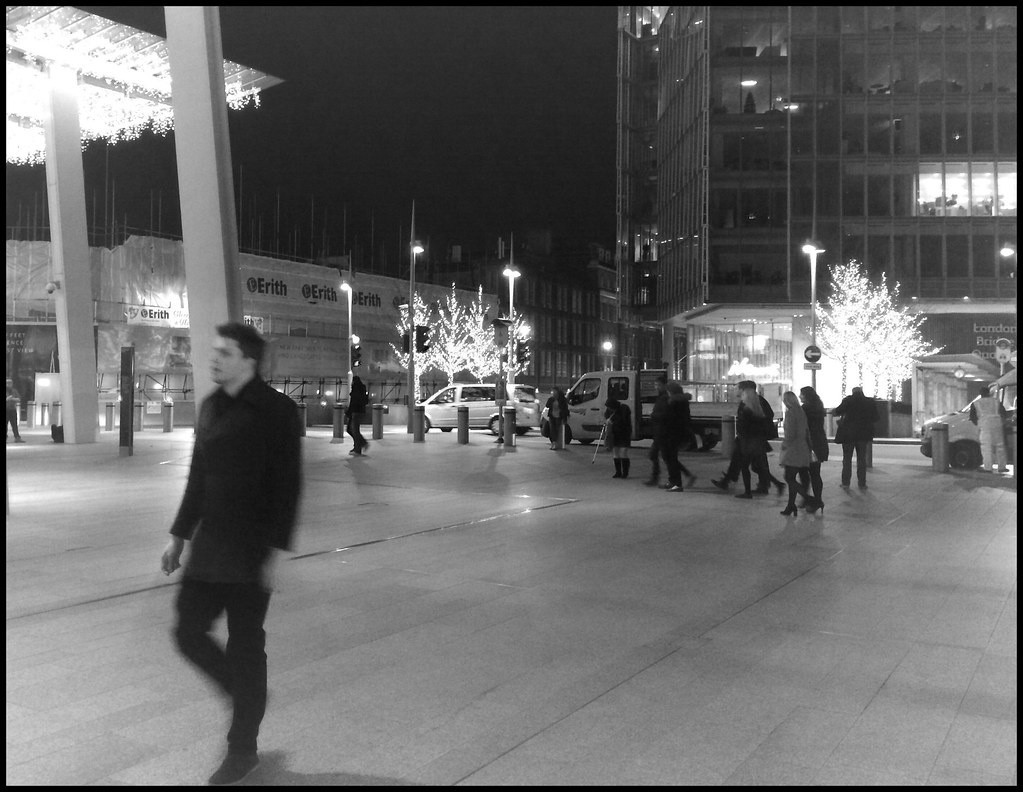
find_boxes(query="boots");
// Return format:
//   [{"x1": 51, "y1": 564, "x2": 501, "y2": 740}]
[
  {"x1": 613, "y1": 458, "x2": 622, "y2": 478},
  {"x1": 621, "y1": 458, "x2": 630, "y2": 478}
]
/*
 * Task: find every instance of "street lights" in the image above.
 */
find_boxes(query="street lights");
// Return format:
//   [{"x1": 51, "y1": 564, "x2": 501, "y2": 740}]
[
  {"x1": 800, "y1": 240, "x2": 826, "y2": 392},
  {"x1": 340, "y1": 283, "x2": 353, "y2": 409},
  {"x1": 602, "y1": 340, "x2": 613, "y2": 372},
  {"x1": 502, "y1": 231, "x2": 521, "y2": 406},
  {"x1": 408, "y1": 199, "x2": 425, "y2": 433}
]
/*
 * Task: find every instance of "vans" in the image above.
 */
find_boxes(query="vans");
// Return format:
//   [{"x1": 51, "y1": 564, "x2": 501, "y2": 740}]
[
  {"x1": 921, "y1": 382, "x2": 1017, "y2": 470},
  {"x1": 418, "y1": 383, "x2": 541, "y2": 436}
]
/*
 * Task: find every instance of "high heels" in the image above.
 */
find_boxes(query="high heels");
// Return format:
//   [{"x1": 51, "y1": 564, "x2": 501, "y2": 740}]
[
  {"x1": 806, "y1": 501, "x2": 824, "y2": 513},
  {"x1": 780, "y1": 504, "x2": 797, "y2": 516}
]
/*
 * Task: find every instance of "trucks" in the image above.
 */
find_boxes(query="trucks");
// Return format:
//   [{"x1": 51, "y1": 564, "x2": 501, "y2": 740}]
[{"x1": 541, "y1": 369, "x2": 739, "y2": 452}]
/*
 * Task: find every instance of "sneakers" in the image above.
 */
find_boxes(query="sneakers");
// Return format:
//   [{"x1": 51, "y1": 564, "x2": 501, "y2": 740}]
[{"x1": 209, "y1": 753, "x2": 261, "y2": 784}]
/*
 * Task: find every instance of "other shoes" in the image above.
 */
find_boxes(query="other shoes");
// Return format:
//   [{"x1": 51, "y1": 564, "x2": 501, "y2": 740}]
[
  {"x1": 978, "y1": 469, "x2": 992, "y2": 473},
  {"x1": 550, "y1": 442, "x2": 558, "y2": 449},
  {"x1": 667, "y1": 485, "x2": 683, "y2": 492},
  {"x1": 752, "y1": 488, "x2": 767, "y2": 494},
  {"x1": 659, "y1": 481, "x2": 671, "y2": 489},
  {"x1": 840, "y1": 485, "x2": 848, "y2": 488},
  {"x1": 859, "y1": 485, "x2": 868, "y2": 488},
  {"x1": 998, "y1": 469, "x2": 1008, "y2": 472},
  {"x1": 736, "y1": 493, "x2": 751, "y2": 498},
  {"x1": 778, "y1": 484, "x2": 785, "y2": 496},
  {"x1": 711, "y1": 479, "x2": 725, "y2": 488}
]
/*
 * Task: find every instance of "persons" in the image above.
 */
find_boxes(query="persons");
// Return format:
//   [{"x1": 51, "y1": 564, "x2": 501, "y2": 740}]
[
  {"x1": 970, "y1": 387, "x2": 1010, "y2": 473},
  {"x1": 603, "y1": 397, "x2": 632, "y2": 479},
  {"x1": 161, "y1": 322, "x2": 304, "y2": 785},
  {"x1": 779, "y1": 386, "x2": 829, "y2": 516},
  {"x1": 346, "y1": 375, "x2": 369, "y2": 454},
  {"x1": 826, "y1": 386, "x2": 879, "y2": 490},
  {"x1": 711, "y1": 380, "x2": 786, "y2": 498},
  {"x1": 545, "y1": 387, "x2": 570, "y2": 450},
  {"x1": 6, "y1": 376, "x2": 26, "y2": 443},
  {"x1": 659, "y1": 380, "x2": 692, "y2": 492},
  {"x1": 642, "y1": 376, "x2": 697, "y2": 489}
]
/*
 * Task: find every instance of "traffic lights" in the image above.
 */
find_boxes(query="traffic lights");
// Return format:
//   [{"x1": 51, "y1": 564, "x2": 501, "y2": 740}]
[
  {"x1": 416, "y1": 325, "x2": 430, "y2": 353},
  {"x1": 351, "y1": 345, "x2": 361, "y2": 368}
]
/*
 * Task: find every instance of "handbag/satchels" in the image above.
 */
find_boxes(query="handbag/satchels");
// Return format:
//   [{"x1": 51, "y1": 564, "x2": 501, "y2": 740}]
[{"x1": 809, "y1": 450, "x2": 819, "y2": 463}]
